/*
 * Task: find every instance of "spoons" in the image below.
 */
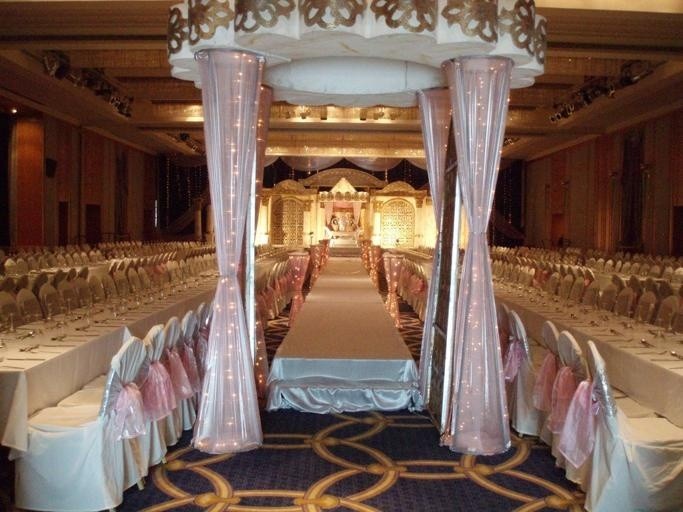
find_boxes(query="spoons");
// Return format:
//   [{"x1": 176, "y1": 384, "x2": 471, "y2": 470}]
[
  {"x1": 668, "y1": 349, "x2": 683, "y2": 361},
  {"x1": 641, "y1": 339, "x2": 656, "y2": 348}
]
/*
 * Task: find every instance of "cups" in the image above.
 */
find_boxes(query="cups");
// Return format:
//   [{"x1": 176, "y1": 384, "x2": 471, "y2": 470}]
[
  {"x1": 0, "y1": 267, "x2": 215, "y2": 382},
  {"x1": 493, "y1": 262, "x2": 683, "y2": 361}
]
